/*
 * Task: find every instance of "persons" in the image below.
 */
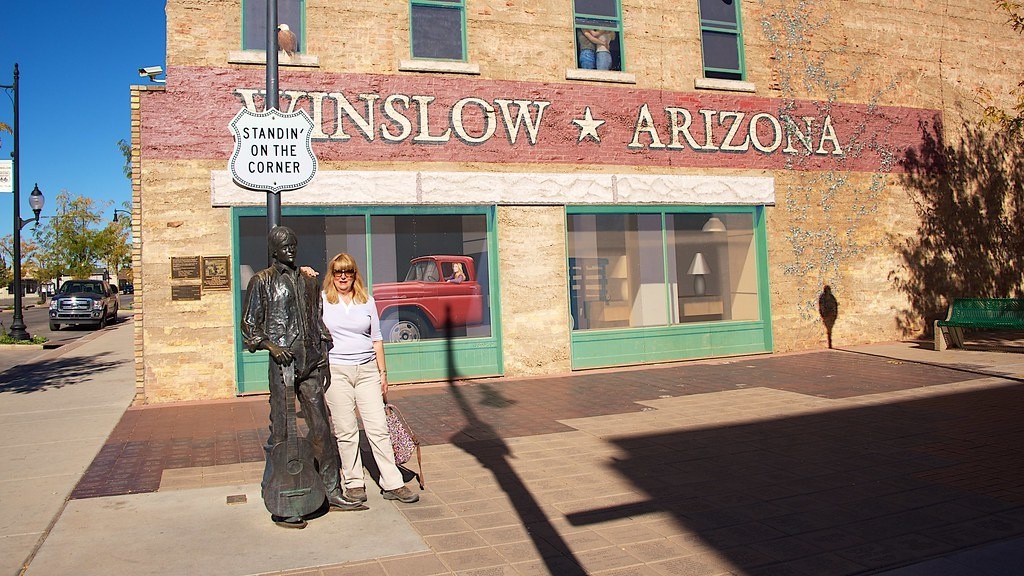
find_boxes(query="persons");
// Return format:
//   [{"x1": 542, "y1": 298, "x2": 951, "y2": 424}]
[
  {"x1": 444, "y1": 263, "x2": 466, "y2": 284},
  {"x1": 241, "y1": 226, "x2": 363, "y2": 528},
  {"x1": 301, "y1": 253, "x2": 419, "y2": 502},
  {"x1": 577, "y1": 19, "x2": 616, "y2": 71}
]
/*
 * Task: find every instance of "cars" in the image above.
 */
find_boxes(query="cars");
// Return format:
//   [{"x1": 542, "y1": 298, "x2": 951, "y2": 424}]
[{"x1": 124, "y1": 284, "x2": 134, "y2": 294}]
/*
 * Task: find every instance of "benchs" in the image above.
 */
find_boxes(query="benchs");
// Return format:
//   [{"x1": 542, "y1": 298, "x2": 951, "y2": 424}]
[{"x1": 934, "y1": 297, "x2": 1024, "y2": 351}]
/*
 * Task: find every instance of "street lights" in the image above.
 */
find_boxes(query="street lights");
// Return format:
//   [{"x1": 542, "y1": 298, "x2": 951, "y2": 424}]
[{"x1": 4, "y1": 184, "x2": 46, "y2": 340}]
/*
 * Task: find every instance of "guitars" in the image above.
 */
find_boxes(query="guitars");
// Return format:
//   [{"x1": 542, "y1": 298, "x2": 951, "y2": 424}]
[{"x1": 263, "y1": 355, "x2": 325, "y2": 517}]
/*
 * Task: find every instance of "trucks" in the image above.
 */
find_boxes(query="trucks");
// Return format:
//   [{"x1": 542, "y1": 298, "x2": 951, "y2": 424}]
[{"x1": 367, "y1": 254, "x2": 611, "y2": 342}]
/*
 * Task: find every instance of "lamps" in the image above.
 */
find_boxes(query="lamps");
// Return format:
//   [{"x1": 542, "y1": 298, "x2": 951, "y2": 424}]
[
  {"x1": 686, "y1": 252, "x2": 712, "y2": 295},
  {"x1": 608, "y1": 255, "x2": 628, "y2": 300},
  {"x1": 702, "y1": 214, "x2": 727, "y2": 232}
]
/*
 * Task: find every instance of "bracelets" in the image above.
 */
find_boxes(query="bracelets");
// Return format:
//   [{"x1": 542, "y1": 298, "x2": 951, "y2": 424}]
[{"x1": 380, "y1": 370, "x2": 387, "y2": 376}]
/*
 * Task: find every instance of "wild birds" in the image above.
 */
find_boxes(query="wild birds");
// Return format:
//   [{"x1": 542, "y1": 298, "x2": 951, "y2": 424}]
[{"x1": 277, "y1": 23, "x2": 297, "y2": 59}]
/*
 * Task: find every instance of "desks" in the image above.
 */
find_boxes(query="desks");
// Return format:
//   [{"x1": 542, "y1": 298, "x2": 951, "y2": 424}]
[{"x1": 583, "y1": 293, "x2": 724, "y2": 329}]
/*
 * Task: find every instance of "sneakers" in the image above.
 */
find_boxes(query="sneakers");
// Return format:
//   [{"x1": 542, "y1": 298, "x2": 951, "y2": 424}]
[
  {"x1": 345, "y1": 486, "x2": 367, "y2": 500},
  {"x1": 382, "y1": 486, "x2": 419, "y2": 503}
]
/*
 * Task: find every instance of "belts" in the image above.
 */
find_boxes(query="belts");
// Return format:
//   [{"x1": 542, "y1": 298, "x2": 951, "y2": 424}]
[
  {"x1": 581, "y1": 49, "x2": 593, "y2": 51},
  {"x1": 596, "y1": 51, "x2": 610, "y2": 53}
]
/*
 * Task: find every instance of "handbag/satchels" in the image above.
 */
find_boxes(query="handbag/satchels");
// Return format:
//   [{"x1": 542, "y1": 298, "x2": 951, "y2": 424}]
[{"x1": 382, "y1": 392, "x2": 420, "y2": 465}]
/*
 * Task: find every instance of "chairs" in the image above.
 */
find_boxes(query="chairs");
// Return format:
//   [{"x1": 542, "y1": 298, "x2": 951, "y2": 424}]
[
  {"x1": 71, "y1": 285, "x2": 80, "y2": 293},
  {"x1": 86, "y1": 286, "x2": 92, "y2": 292},
  {"x1": 94, "y1": 285, "x2": 101, "y2": 293}
]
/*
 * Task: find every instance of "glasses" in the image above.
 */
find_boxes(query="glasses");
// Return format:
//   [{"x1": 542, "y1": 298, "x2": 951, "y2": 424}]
[{"x1": 332, "y1": 269, "x2": 355, "y2": 277}]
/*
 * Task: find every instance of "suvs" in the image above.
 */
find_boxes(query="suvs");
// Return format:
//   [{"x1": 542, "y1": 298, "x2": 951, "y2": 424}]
[{"x1": 49, "y1": 279, "x2": 119, "y2": 331}]
